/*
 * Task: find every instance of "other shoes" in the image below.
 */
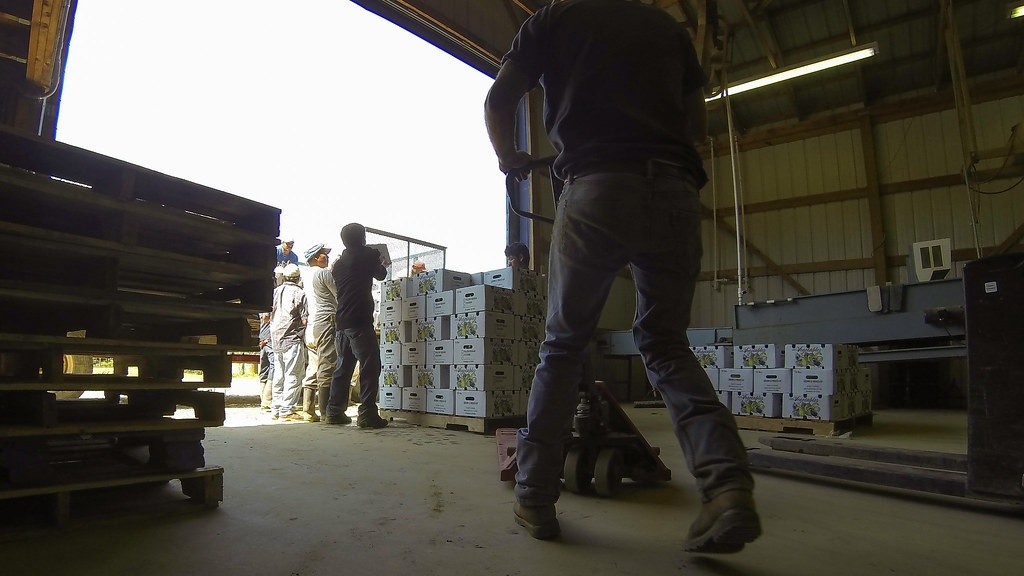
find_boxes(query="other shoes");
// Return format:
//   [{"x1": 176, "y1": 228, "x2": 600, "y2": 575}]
[
  {"x1": 681, "y1": 488, "x2": 763, "y2": 554},
  {"x1": 357, "y1": 416, "x2": 388, "y2": 429},
  {"x1": 325, "y1": 413, "x2": 352, "y2": 424},
  {"x1": 513, "y1": 501, "x2": 560, "y2": 541}
]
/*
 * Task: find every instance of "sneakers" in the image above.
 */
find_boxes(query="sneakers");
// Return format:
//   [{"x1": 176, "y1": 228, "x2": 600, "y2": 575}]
[
  {"x1": 279, "y1": 412, "x2": 304, "y2": 422},
  {"x1": 272, "y1": 405, "x2": 279, "y2": 420}
]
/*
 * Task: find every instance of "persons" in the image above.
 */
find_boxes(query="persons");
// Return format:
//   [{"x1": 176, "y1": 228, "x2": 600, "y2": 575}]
[
  {"x1": 484, "y1": 0, "x2": 763, "y2": 555},
  {"x1": 259, "y1": 312, "x2": 275, "y2": 408},
  {"x1": 505, "y1": 242, "x2": 530, "y2": 270},
  {"x1": 277, "y1": 236, "x2": 298, "y2": 266},
  {"x1": 270, "y1": 262, "x2": 310, "y2": 420},
  {"x1": 302, "y1": 242, "x2": 339, "y2": 423},
  {"x1": 324, "y1": 223, "x2": 389, "y2": 429}
]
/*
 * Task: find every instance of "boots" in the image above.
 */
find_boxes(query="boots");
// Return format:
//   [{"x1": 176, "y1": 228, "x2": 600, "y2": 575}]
[
  {"x1": 318, "y1": 387, "x2": 331, "y2": 422},
  {"x1": 303, "y1": 387, "x2": 320, "y2": 422},
  {"x1": 259, "y1": 380, "x2": 273, "y2": 412}
]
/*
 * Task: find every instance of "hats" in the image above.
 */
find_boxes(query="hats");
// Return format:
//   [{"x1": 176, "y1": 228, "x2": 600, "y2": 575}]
[
  {"x1": 304, "y1": 243, "x2": 332, "y2": 266},
  {"x1": 283, "y1": 264, "x2": 300, "y2": 277},
  {"x1": 281, "y1": 237, "x2": 294, "y2": 244}
]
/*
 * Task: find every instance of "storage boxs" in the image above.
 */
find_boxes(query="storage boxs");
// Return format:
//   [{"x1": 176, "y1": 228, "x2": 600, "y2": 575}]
[
  {"x1": 689, "y1": 344, "x2": 871, "y2": 420},
  {"x1": 376, "y1": 265, "x2": 547, "y2": 419}
]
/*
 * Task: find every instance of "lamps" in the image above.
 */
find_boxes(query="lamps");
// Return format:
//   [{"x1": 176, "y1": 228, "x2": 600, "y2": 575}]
[
  {"x1": 1005, "y1": 0, "x2": 1024, "y2": 22},
  {"x1": 705, "y1": 40, "x2": 879, "y2": 103}
]
